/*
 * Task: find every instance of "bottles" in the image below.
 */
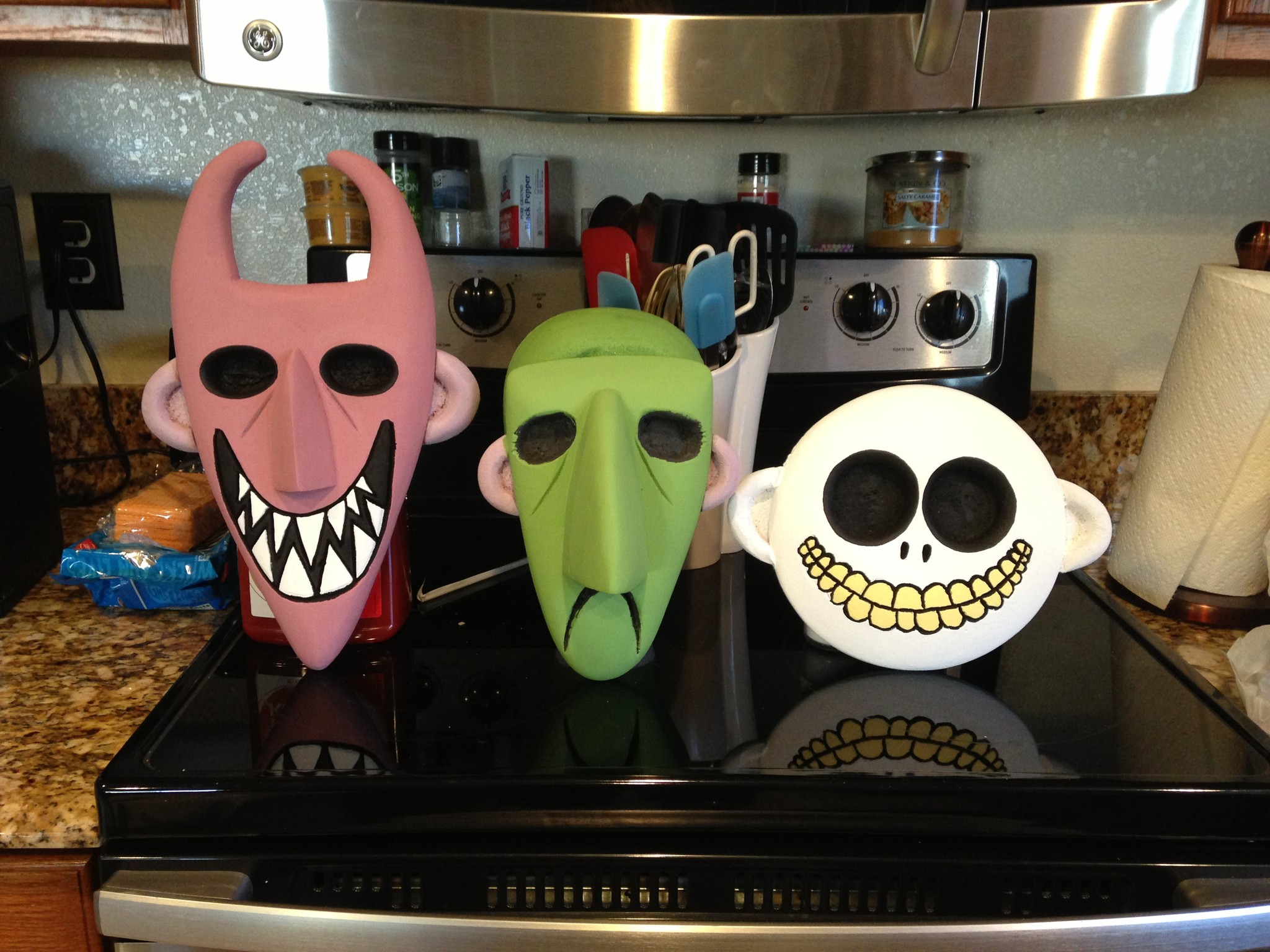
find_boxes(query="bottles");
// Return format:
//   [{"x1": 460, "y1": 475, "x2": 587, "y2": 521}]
[
  {"x1": 429, "y1": 136, "x2": 473, "y2": 248},
  {"x1": 737, "y1": 152, "x2": 781, "y2": 207},
  {"x1": 372, "y1": 130, "x2": 424, "y2": 247}
]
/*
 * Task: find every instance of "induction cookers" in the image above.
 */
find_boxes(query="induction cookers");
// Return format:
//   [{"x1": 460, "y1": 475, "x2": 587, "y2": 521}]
[{"x1": 95, "y1": 250, "x2": 1270, "y2": 879}]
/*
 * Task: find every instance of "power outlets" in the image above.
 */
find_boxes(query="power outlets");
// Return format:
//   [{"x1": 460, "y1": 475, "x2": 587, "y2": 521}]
[{"x1": 30, "y1": 191, "x2": 125, "y2": 311}]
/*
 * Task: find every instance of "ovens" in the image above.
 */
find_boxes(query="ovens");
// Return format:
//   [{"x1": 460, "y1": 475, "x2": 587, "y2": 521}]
[{"x1": 191, "y1": 0, "x2": 1208, "y2": 116}]
